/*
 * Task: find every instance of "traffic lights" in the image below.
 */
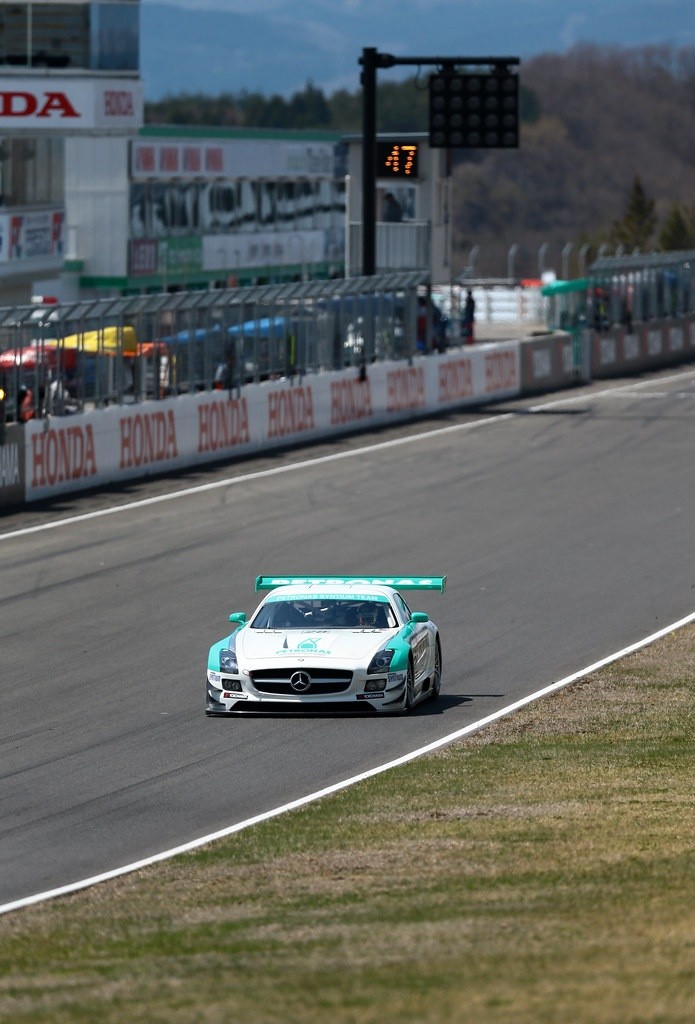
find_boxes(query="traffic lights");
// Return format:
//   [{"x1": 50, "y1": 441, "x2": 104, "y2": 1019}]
[{"x1": 376, "y1": 141, "x2": 419, "y2": 177}]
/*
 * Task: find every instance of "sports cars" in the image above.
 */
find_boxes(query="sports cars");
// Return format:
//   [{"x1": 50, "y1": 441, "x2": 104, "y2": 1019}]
[{"x1": 207, "y1": 574, "x2": 446, "y2": 719}]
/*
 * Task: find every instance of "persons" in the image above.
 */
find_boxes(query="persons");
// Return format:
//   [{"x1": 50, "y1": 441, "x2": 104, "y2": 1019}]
[
  {"x1": 380, "y1": 193, "x2": 404, "y2": 222},
  {"x1": 357, "y1": 603, "x2": 380, "y2": 626},
  {"x1": 16, "y1": 371, "x2": 84, "y2": 422},
  {"x1": 198, "y1": 288, "x2": 478, "y2": 392}
]
